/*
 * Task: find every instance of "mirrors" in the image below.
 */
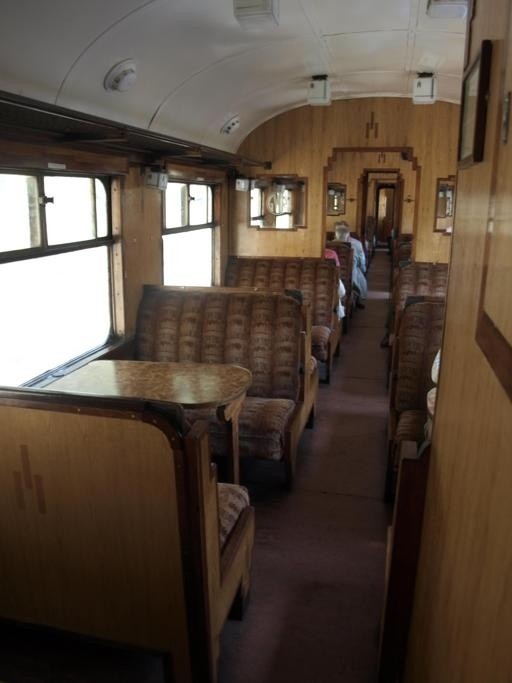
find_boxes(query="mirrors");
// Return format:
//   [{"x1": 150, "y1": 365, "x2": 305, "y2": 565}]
[
  {"x1": 246, "y1": 173, "x2": 308, "y2": 233},
  {"x1": 326, "y1": 182, "x2": 347, "y2": 216},
  {"x1": 434, "y1": 176, "x2": 455, "y2": 234}
]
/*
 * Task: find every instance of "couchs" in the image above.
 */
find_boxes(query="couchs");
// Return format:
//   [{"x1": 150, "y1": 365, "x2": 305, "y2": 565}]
[{"x1": 382, "y1": 233, "x2": 450, "y2": 682}]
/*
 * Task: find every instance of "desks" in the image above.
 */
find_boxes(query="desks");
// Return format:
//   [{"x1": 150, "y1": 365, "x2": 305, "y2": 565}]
[{"x1": 47, "y1": 357, "x2": 254, "y2": 486}]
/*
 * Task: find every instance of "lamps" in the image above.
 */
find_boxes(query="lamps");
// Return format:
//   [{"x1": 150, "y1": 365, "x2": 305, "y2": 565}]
[
  {"x1": 140, "y1": 162, "x2": 168, "y2": 192},
  {"x1": 307, "y1": 74, "x2": 332, "y2": 107},
  {"x1": 413, "y1": 72, "x2": 437, "y2": 105}
]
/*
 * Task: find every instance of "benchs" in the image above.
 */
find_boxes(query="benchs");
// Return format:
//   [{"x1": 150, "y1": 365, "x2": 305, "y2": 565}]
[
  {"x1": 1, "y1": 384, "x2": 257, "y2": 683},
  {"x1": 328, "y1": 241, "x2": 354, "y2": 331},
  {"x1": 226, "y1": 254, "x2": 343, "y2": 383},
  {"x1": 130, "y1": 282, "x2": 316, "y2": 485}
]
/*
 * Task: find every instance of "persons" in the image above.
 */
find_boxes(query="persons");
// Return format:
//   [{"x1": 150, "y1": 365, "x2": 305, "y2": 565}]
[{"x1": 330, "y1": 219, "x2": 367, "y2": 309}]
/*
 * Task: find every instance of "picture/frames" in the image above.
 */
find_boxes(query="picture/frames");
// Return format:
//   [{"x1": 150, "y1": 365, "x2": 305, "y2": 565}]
[{"x1": 457, "y1": 39, "x2": 493, "y2": 169}]
